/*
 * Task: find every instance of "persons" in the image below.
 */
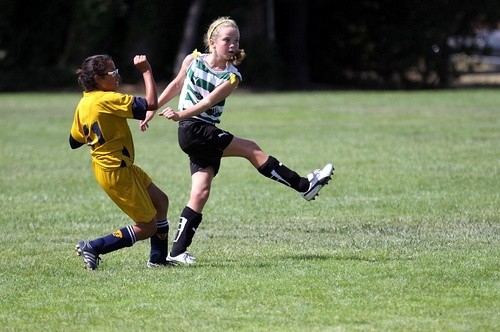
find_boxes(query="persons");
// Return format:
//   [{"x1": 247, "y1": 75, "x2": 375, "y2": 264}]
[
  {"x1": 69, "y1": 54, "x2": 185, "y2": 269},
  {"x1": 139, "y1": 15, "x2": 335, "y2": 266}
]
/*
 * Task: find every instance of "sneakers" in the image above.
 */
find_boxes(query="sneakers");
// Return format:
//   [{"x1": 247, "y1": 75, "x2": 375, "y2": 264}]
[
  {"x1": 166, "y1": 251, "x2": 197, "y2": 265},
  {"x1": 147, "y1": 260, "x2": 182, "y2": 269},
  {"x1": 75, "y1": 240, "x2": 101, "y2": 272},
  {"x1": 300, "y1": 163, "x2": 334, "y2": 202}
]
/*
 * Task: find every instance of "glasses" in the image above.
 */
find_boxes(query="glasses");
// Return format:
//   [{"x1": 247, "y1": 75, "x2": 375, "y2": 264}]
[{"x1": 101, "y1": 69, "x2": 118, "y2": 77}]
